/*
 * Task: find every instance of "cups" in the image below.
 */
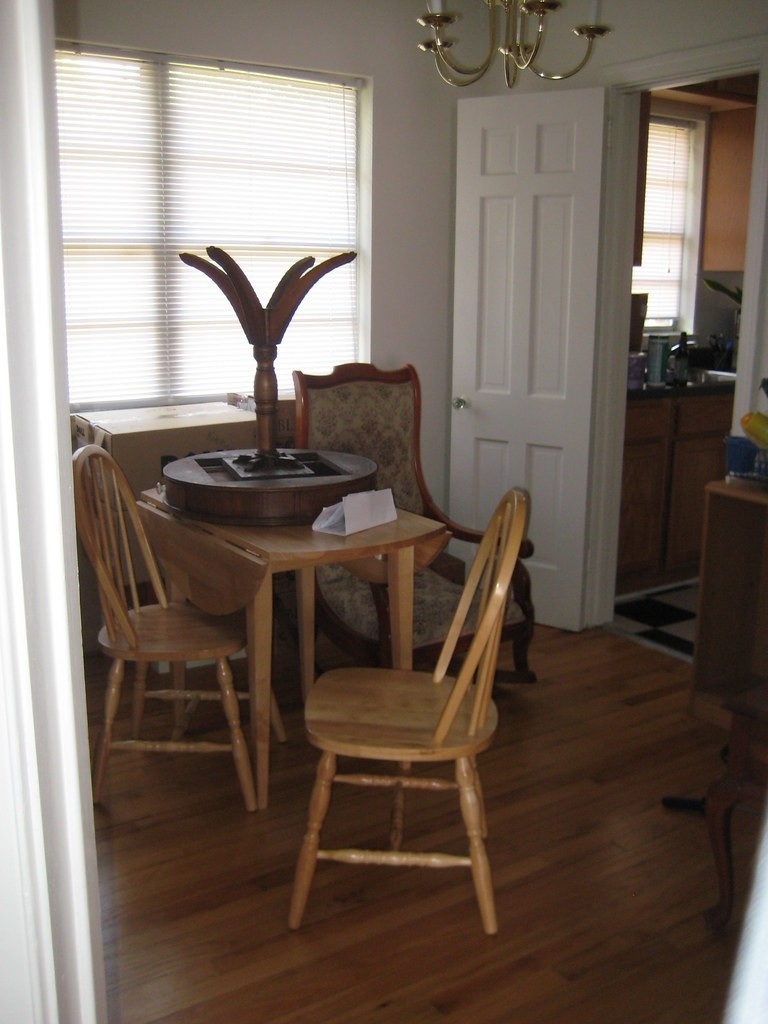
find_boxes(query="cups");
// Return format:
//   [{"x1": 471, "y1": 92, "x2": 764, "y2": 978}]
[{"x1": 628, "y1": 351, "x2": 647, "y2": 389}]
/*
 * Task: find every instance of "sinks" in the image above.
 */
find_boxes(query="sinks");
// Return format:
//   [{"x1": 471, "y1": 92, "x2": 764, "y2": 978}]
[{"x1": 685, "y1": 366, "x2": 737, "y2": 389}]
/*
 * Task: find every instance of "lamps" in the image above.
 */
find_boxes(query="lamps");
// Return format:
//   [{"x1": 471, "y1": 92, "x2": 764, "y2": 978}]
[{"x1": 417, "y1": 0, "x2": 611, "y2": 88}]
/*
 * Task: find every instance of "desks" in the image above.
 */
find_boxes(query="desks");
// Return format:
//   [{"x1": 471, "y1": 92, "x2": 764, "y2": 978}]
[
  {"x1": 688, "y1": 477, "x2": 768, "y2": 715},
  {"x1": 135, "y1": 482, "x2": 453, "y2": 703}
]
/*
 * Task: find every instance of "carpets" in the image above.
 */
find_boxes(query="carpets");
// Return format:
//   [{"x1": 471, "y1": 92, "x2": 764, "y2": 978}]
[{"x1": 602, "y1": 586, "x2": 696, "y2": 664}]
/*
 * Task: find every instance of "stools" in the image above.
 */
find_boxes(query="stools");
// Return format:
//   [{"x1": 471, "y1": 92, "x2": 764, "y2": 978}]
[{"x1": 703, "y1": 681, "x2": 768, "y2": 929}]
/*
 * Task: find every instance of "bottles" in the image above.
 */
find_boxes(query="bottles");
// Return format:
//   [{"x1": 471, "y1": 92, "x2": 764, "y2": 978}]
[{"x1": 673, "y1": 332, "x2": 690, "y2": 387}]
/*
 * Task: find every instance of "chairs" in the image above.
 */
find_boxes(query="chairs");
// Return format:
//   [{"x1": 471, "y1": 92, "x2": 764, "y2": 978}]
[
  {"x1": 73, "y1": 444, "x2": 286, "y2": 813},
  {"x1": 293, "y1": 362, "x2": 537, "y2": 685},
  {"x1": 284, "y1": 488, "x2": 528, "y2": 936}
]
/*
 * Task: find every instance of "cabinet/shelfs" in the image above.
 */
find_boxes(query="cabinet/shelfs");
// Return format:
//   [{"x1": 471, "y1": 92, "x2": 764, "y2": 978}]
[
  {"x1": 633, "y1": 74, "x2": 758, "y2": 272},
  {"x1": 615, "y1": 393, "x2": 734, "y2": 597}
]
